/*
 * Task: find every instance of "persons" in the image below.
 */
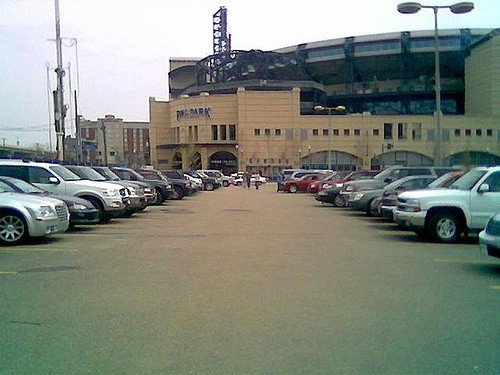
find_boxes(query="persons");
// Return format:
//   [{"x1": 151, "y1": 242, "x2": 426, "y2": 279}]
[
  {"x1": 276, "y1": 172, "x2": 285, "y2": 192},
  {"x1": 243, "y1": 169, "x2": 251, "y2": 189},
  {"x1": 254, "y1": 171, "x2": 260, "y2": 190}
]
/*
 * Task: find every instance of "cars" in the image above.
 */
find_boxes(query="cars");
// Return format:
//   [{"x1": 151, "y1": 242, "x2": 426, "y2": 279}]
[
  {"x1": 63, "y1": 165, "x2": 147, "y2": 217},
  {"x1": 278, "y1": 174, "x2": 321, "y2": 194},
  {"x1": 92, "y1": 162, "x2": 268, "y2": 210},
  {"x1": 0, "y1": 181, "x2": 70, "y2": 245},
  {"x1": 1, "y1": 176, "x2": 100, "y2": 223},
  {"x1": 281, "y1": 167, "x2": 464, "y2": 229}
]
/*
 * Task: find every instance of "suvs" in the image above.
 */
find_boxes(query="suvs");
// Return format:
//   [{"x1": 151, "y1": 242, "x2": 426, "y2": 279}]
[
  {"x1": 0, "y1": 159, "x2": 129, "y2": 221},
  {"x1": 393, "y1": 166, "x2": 500, "y2": 243}
]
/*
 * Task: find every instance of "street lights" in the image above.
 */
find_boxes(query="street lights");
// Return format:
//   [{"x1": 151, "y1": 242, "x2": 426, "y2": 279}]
[
  {"x1": 308, "y1": 145, "x2": 312, "y2": 169},
  {"x1": 314, "y1": 105, "x2": 345, "y2": 171},
  {"x1": 396, "y1": 1, "x2": 474, "y2": 168}
]
